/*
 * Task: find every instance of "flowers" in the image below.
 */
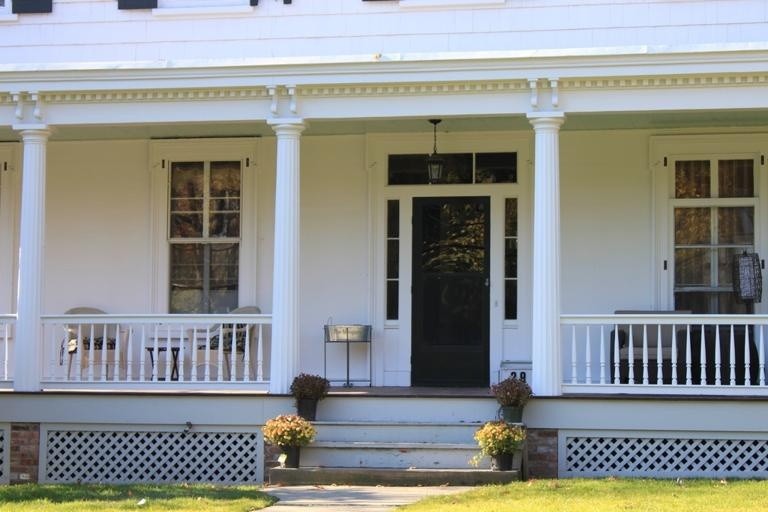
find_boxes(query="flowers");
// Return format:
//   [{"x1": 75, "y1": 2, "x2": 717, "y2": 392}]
[
  {"x1": 262, "y1": 414, "x2": 316, "y2": 446},
  {"x1": 291, "y1": 372, "x2": 330, "y2": 401},
  {"x1": 490, "y1": 377, "x2": 535, "y2": 404},
  {"x1": 468, "y1": 420, "x2": 528, "y2": 469}
]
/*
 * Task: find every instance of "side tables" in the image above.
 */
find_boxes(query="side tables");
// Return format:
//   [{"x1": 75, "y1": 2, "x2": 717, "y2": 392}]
[{"x1": 144, "y1": 347, "x2": 181, "y2": 380}]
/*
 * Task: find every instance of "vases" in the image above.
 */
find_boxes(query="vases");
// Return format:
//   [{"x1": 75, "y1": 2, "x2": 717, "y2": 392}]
[
  {"x1": 280, "y1": 445, "x2": 300, "y2": 467},
  {"x1": 489, "y1": 452, "x2": 513, "y2": 471},
  {"x1": 502, "y1": 406, "x2": 523, "y2": 421},
  {"x1": 295, "y1": 398, "x2": 317, "y2": 420}
]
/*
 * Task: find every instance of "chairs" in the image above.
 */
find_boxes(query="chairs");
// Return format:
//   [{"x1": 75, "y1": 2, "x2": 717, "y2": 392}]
[
  {"x1": 611, "y1": 310, "x2": 694, "y2": 383},
  {"x1": 65, "y1": 306, "x2": 133, "y2": 380},
  {"x1": 187, "y1": 306, "x2": 261, "y2": 381}
]
